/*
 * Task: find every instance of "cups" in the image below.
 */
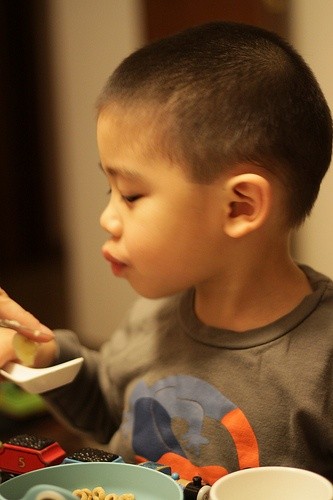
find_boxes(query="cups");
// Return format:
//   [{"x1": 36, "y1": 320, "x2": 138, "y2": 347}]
[{"x1": 210, "y1": 466, "x2": 333, "y2": 500}]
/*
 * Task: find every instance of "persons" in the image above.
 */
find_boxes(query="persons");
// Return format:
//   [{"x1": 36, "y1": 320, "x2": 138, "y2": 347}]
[{"x1": 0, "y1": 21, "x2": 333, "y2": 486}]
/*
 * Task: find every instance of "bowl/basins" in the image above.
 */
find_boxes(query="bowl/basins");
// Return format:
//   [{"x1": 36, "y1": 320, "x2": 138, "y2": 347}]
[{"x1": 0, "y1": 462, "x2": 183, "y2": 500}]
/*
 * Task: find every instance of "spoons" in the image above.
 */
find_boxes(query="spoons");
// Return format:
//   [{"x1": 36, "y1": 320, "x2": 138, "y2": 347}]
[{"x1": 0, "y1": 357, "x2": 84, "y2": 394}]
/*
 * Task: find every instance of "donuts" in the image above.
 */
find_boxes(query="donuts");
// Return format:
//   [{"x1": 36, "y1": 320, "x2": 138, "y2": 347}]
[{"x1": 72, "y1": 487, "x2": 135, "y2": 500}]
